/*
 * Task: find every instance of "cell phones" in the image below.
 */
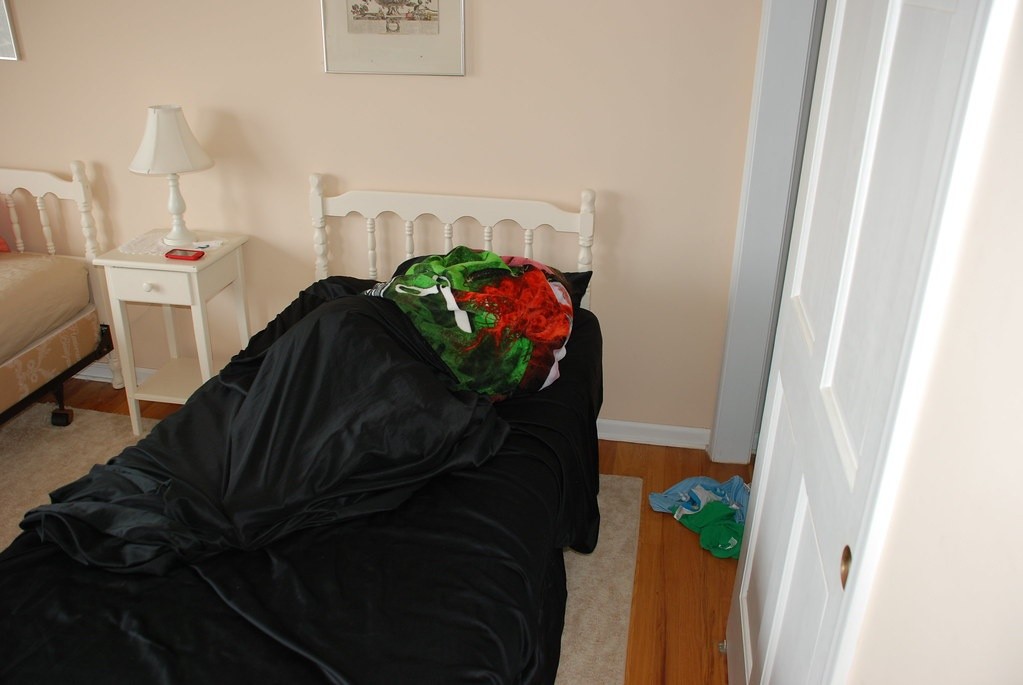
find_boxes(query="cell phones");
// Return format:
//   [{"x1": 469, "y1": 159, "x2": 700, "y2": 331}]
[{"x1": 165, "y1": 249, "x2": 204, "y2": 261}]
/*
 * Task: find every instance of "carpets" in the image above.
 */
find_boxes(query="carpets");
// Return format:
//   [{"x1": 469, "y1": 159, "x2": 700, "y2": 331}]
[{"x1": 0, "y1": 406, "x2": 644, "y2": 685}]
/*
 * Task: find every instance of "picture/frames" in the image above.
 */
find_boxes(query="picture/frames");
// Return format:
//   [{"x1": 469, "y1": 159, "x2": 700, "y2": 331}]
[
  {"x1": 0, "y1": 0, "x2": 20, "y2": 62},
  {"x1": 320, "y1": 0, "x2": 466, "y2": 75}
]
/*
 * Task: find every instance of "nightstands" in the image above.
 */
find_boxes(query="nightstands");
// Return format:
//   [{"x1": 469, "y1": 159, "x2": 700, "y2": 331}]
[{"x1": 92, "y1": 234, "x2": 250, "y2": 436}]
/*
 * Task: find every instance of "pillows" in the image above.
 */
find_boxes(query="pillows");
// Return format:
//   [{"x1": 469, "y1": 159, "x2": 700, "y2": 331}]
[{"x1": 391, "y1": 254, "x2": 595, "y2": 321}]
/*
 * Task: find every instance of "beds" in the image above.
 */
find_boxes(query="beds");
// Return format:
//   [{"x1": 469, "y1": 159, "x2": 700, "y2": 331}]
[{"x1": 0, "y1": 160, "x2": 602, "y2": 685}]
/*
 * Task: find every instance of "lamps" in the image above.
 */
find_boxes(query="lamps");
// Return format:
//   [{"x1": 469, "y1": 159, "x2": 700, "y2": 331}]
[{"x1": 128, "y1": 105, "x2": 215, "y2": 244}]
